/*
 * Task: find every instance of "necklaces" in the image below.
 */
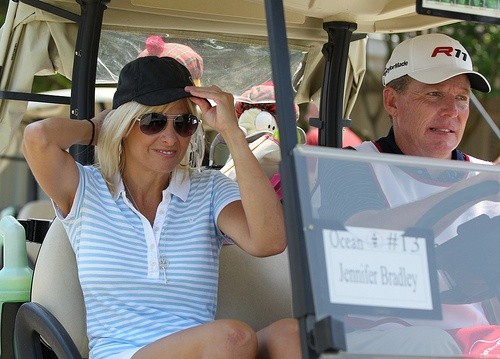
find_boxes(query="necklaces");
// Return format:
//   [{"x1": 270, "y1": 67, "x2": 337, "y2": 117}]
[{"x1": 122, "y1": 176, "x2": 168, "y2": 285}]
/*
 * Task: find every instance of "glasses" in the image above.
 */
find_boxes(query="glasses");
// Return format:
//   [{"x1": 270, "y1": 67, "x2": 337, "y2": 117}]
[{"x1": 132, "y1": 110, "x2": 204, "y2": 138}]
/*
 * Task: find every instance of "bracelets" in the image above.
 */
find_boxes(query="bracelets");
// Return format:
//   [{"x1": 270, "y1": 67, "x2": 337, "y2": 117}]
[{"x1": 85, "y1": 119, "x2": 95, "y2": 147}]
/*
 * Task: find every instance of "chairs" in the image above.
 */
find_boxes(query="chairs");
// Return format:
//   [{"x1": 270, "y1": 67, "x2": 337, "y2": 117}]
[{"x1": 0, "y1": 120, "x2": 275, "y2": 359}]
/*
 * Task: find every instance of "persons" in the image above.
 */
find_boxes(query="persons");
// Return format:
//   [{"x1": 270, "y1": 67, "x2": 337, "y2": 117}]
[
  {"x1": 299, "y1": 34, "x2": 500, "y2": 359},
  {"x1": 20, "y1": 57, "x2": 303, "y2": 359}
]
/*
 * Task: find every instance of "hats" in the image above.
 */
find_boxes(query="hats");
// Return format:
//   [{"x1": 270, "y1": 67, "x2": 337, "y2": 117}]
[
  {"x1": 111, "y1": 55, "x2": 214, "y2": 111},
  {"x1": 136, "y1": 35, "x2": 205, "y2": 88},
  {"x1": 382, "y1": 32, "x2": 493, "y2": 93}
]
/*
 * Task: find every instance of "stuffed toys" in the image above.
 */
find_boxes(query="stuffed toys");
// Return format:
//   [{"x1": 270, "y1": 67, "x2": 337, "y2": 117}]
[{"x1": 235, "y1": 82, "x2": 300, "y2": 144}]
